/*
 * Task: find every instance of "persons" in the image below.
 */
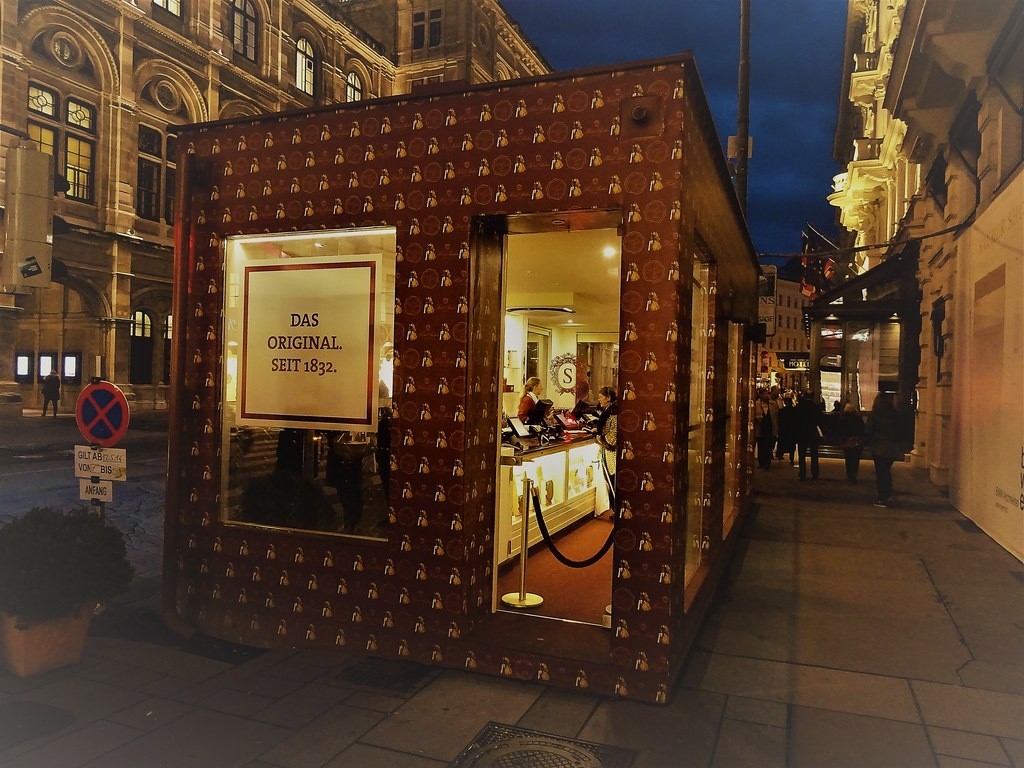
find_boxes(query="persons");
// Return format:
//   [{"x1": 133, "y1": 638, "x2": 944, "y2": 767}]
[
  {"x1": 755, "y1": 386, "x2": 905, "y2": 508},
  {"x1": 518, "y1": 377, "x2": 543, "y2": 423},
  {"x1": 326, "y1": 398, "x2": 392, "y2": 535},
  {"x1": 592, "y1": 387, "x2": 617, "y2": 519},
  {"x1": 41, "y1": 370, "x2": 60, "y2": 416}
]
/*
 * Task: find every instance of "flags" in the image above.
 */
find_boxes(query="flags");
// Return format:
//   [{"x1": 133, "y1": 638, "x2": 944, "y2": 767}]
[{"x1": 799, "y1": 225, "x2": 840, "y2": 299}]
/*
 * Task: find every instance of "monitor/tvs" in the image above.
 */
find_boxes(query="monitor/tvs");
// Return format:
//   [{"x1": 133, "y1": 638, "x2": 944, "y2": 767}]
[
  {"x1": 526, "y1": 398, "x2": 553, "y2": 423},
  {"x1": 571, "y1": 399, "x2": 589, "y2": 420}
]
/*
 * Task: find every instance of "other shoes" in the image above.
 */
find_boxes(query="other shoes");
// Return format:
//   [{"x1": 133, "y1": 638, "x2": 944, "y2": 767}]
[
  {"x1": 597, "y1": 509, "x2": 615, "y2": 520},
  {"x1": 872, "y1": 500, "x2": 887, "y2": 507}
]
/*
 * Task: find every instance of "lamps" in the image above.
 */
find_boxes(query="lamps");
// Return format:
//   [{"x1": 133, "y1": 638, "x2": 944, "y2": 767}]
[{"x1": 18, "y1": 258, "x2": 42, "y2": 278}]
